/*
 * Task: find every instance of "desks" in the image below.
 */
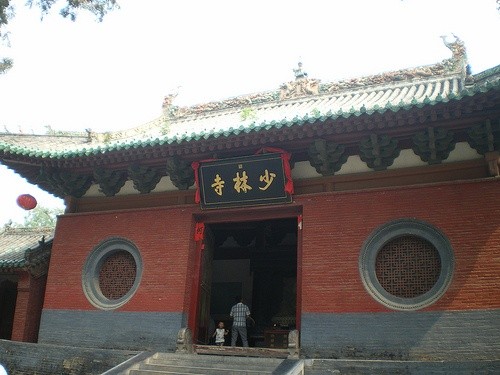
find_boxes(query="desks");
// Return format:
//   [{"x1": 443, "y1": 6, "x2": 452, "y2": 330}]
[{"x1": 264, "y1": 330, "x2": 289, "y2": 348}]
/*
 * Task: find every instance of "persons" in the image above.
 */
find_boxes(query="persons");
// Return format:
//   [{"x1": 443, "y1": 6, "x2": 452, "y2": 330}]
[
  {"x1": 229, "y1": 296, "x2": 250, "y2": 347},
  {"x1": 211, "y1": 322, "x2": 229, "y2": 346}
]
